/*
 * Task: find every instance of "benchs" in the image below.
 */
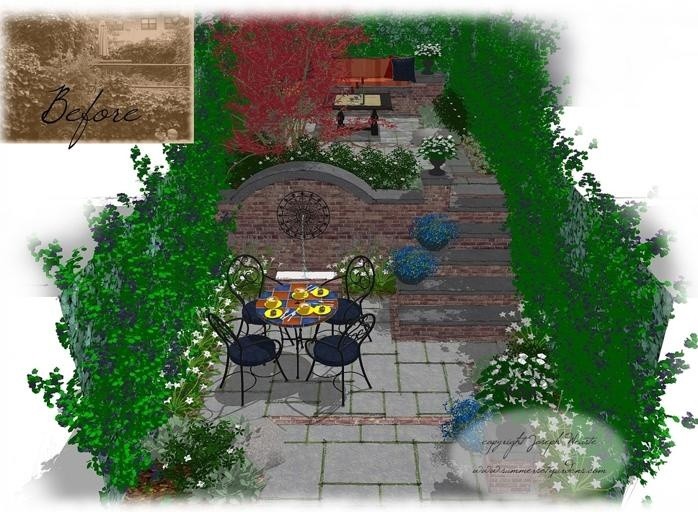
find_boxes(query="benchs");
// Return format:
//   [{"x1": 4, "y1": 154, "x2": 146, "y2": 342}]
[{"x1": 322, "y1": 58, "x2": 415, "y2": 118}]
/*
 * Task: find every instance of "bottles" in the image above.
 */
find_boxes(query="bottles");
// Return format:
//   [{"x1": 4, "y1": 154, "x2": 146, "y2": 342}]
[{"x1": 371, "y1": 109, "x2": 378, "y2": 136}]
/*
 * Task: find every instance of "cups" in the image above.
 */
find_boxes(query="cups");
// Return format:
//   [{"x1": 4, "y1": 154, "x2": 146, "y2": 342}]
[
  {"x1": 300, "y1": 302, "x2": 311, "y2": 312},
  {"x1": 295, "y1": 287, "x2": 305, "y2": 297},
  {"x1": 266, "y1": 296, "x2": 278, "y2": 306}
]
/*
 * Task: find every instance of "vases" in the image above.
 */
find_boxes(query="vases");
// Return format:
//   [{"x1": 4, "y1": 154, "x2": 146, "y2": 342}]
[
  {"x1": 420, "y1": 59, "x2": 435, "y2": 76},
  {"x1": 427, "y1": 159, "x2": 448, "y2": 177}
]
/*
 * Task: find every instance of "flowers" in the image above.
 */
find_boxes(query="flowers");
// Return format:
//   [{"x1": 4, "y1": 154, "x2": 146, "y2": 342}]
[
  {"x1": 415, "y1": 131, "x2": 462, "y2": 162},
  {"x1": 414, "y1": 42, "x2": 442, "y2": 59}
]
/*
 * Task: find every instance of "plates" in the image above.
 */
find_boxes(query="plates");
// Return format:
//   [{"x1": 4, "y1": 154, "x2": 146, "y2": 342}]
[
  {"x1": 314, "y1": 305, "x2": 331, "y2": 316},
  {"x1": 264, "y1": 301, "x2": 282, "y2": 309},
  {"x1": 292, "y1": 292, "x2": 309, "y2": 300},
  {"x1": 312, "y1": 288, "x2": 330, "y2": 298},
  {"x1": 265, "y1": 309, "x2": 282, "y2": 319},
  {"x1": 296, "y1": 307, "x2": 314, "y2": 316}
]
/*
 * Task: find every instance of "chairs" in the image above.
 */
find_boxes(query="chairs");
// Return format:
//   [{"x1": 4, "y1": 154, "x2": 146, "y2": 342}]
[{"x1": 206, "y1": 255, "x2": 377, "y2": 406}]
